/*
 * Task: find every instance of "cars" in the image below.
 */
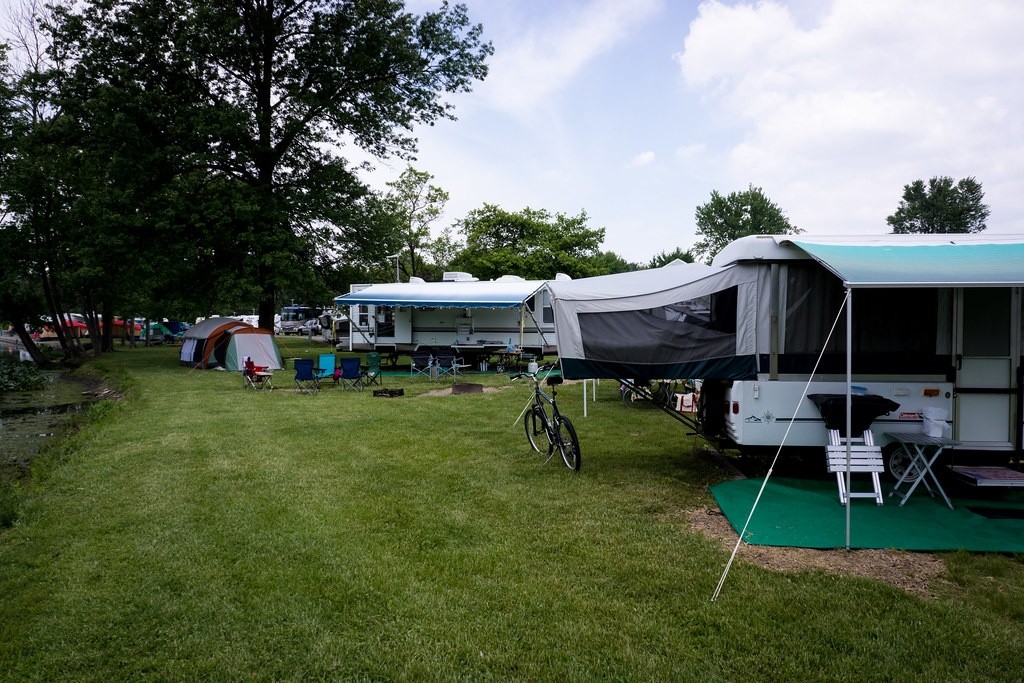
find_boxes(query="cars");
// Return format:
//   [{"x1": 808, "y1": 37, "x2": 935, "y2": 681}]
[
  {"x1": 23, "y1": 311, "x2": 159, "y2": 331},
  {"x1": 299, "y1": 319, "x2": 324, "y2": 337}
]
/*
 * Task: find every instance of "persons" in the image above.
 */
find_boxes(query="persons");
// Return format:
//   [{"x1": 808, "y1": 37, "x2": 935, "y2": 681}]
[
  {"x1": 9, "y1": 325, "x2": 14, "y2": 337},
  {"x1": 32, "y1": 330, "x2": 41, "y2": 339},
  {"x1": 310, "y1": 320, "x2": 320, "y2": 336}
]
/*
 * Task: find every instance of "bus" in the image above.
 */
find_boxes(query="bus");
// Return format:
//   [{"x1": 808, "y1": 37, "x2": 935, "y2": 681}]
[{"x1": 280, "y1": 306, "x2": 330, "y2": 336}]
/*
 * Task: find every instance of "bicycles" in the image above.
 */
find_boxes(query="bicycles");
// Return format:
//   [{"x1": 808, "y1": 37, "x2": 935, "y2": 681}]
[{"x1": 509, "y1": 363, "x2": 582, "y2": 472}]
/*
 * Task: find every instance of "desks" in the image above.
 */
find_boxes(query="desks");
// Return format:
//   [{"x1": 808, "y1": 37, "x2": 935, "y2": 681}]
[
  {"x1": 886, "y1": 433, "x2": 962, "y2": 510},
  {"x1": 825, "y1": 429, "x2": 885, "y2": 508},
  {"x1": 458, "y1": 364, "x2": 472, "y2": 382},
  {"x1": 352, "y1": 366, "x2": 379, "y2": 387},
  {"x1": 256, "y1": 373, "x2": 272, "y2": 390}
]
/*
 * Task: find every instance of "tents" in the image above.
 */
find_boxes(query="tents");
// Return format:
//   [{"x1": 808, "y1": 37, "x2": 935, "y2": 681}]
[
  {"x1": 179, "y1": 317, "x2": 285, "y2": 370},
  {"x1": 41, "y1": 313, "x2": 141, "y2": 337},
  {"x1": 139, "y1": 321, "x2": 189, "y2": 342}
]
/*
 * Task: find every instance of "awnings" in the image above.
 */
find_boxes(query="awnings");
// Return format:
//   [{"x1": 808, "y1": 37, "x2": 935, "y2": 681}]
[
  {"x1": 785, "y1": 236, "x2": 1023, "y2": 549},
  {"x1": 332, "y1": 290, "x2": 548, "y2": 346},
  {"x1": 545, "y1": 265, "x2": 757, "y2": 429}
]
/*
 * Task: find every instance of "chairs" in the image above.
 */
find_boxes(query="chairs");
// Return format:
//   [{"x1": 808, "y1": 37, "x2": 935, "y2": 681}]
[
  {"x1": 435, "y1": 356, "x2": 457, "y2": 383},
  {"x1": 317, "y1": 353, "x2": 341, "y2": 388},
  {"x1": 411, "y1": 356, "x2": 434, "y2": 381},
  {"x1": 294, "y1": 359, "x2": 327, "y2": 396},
  {"x1": 242, "y1": 357, "x2": 274, "y2": 390},
  {"x1": 365, "y1": 352, "x2": 381, "y2": 386},
  {"x1": 340, "y1": 359, "x2": 364, "y2": 391}
]
